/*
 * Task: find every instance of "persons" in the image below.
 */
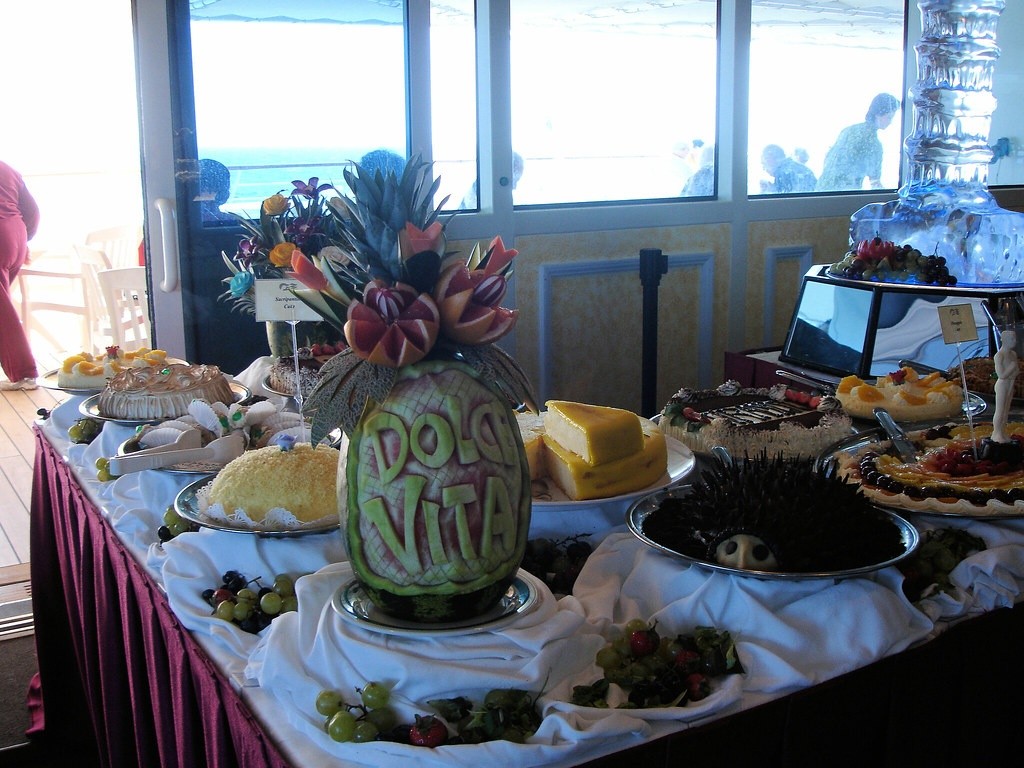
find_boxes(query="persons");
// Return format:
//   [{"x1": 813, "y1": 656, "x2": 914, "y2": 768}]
[
  {"x1": 756, "y1": 143, "x2": 818, "y2": 194},
  {"x1": 811, "y1": 92, "x2": 901, "y2": 194},
  {"x1": 455, "y1": 149, "x2": 524, "y2": 210},
  {"x1": 0, "y1": 161, "x2": 40, "y2": 392},
  {"x1": 194, "y1": 159, "x2": 246, "y2": 222},
  {"x1": 680, "y1": 146, "x2": 714, "y2": 199}
]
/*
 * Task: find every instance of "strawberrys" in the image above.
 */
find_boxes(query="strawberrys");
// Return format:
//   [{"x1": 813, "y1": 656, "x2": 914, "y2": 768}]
[
  {"x1": 676, "y1": 650, "x2": 701, "y2": 667},
  {"x1": 409, "y1": 714, "x2": 448, "y2": 747},
  {"x1": 630, "y1": 618, "x2": 661, "y2": 660},
  {"x1": 685, "y1": 673, "x2": 711, "y2": 701}
]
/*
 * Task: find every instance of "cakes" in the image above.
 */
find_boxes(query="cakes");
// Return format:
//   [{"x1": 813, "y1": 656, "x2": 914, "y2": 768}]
[
  {"x1": 512, "y1": 400, "x2": 668, "y2": 500},
  {"x1": 643, "y1": 356, "x2": 1023, "y2": 574},
  {"x1": 55, "y1": 341, "x2": 353, "y2": 530}
]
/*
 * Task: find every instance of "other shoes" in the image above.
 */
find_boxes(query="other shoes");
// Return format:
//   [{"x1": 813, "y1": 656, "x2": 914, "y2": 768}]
[{"x1": 0, "y1": 377, "x2": 40, "y2": 391}]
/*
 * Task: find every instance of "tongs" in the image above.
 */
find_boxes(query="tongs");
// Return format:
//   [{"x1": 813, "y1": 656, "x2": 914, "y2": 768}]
[{"x1": 111, "y1": 430, "x2": 244, "y2": 477}]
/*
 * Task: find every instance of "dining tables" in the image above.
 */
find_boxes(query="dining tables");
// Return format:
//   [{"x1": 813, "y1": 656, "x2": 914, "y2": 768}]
[{"x1": 30, "y1": 345, "x2": 1024, "y2": 768}]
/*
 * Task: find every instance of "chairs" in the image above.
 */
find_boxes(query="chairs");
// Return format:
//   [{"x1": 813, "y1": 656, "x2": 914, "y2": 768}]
[{"x1": 97, "y1": 266, "x2": 154, "y2": 352}]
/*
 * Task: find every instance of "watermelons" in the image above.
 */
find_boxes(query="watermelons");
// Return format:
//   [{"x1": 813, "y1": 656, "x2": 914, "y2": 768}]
[{"x1": 335, "y1": 359, "x2": 532, "y2": 623}]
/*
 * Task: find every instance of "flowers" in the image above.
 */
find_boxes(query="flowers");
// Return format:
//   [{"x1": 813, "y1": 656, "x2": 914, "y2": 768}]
[
  {"x1": 283, "y1": 154, "x2": 542, "y2": 450},
  {"x1": 217, "y1": 175, "x2": 355, "y2": 314}
]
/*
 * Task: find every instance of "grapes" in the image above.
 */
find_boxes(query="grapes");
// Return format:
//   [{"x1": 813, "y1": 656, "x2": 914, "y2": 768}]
[
  {"x1": 201, "y1": 536, "x2": 711, "y2": 746},
  {"x1": 95, "y1": 457, "x2": 119, "y2": 482},
  {"x1": 827, "y1": 243, "x2": 958, "y2": 286},
  {"x1": 163, "y1": 504, "x2": 197, "y2": 537},
  {"x1": 68, "y1": 417, "x2": 104, "y2": 442}
]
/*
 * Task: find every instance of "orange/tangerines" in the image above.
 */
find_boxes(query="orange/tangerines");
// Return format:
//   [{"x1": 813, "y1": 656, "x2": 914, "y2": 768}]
[{"x1": 345, "y1": 262, "x2": 519, "y2": 366}]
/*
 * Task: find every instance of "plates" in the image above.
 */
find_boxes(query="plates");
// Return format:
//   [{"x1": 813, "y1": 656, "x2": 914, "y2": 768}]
[
  {"x1": 36, "y1": 356, "x2": 190, "y2": 393},
  {"x1": 117, "y1": 438, "x2": 218, "y2": 473},
  {"x1": 262, "y1": 376, "x2": 309, "y2": 401},
  {"x1": 866, "y1": 390, "x2": 987, "y2": 421},
  {"x1": 330, "y1": 568, "x2": 538, "y2": 636},
  {"x1": 626, "y1": 484, "x2": 919, "y2": 578},
  {"x1": 811, "y1": 419, "x2": 1024, "y2": 519},
  {"x1": 175, "y1": 470, "x2": 340, "y2": 536},
  {"x1": 78, "y1": 383, "x2": 253, "y2": 422},
  {"x1": 529, "y1": 431, "x2": 696, "y2": 506}
]
